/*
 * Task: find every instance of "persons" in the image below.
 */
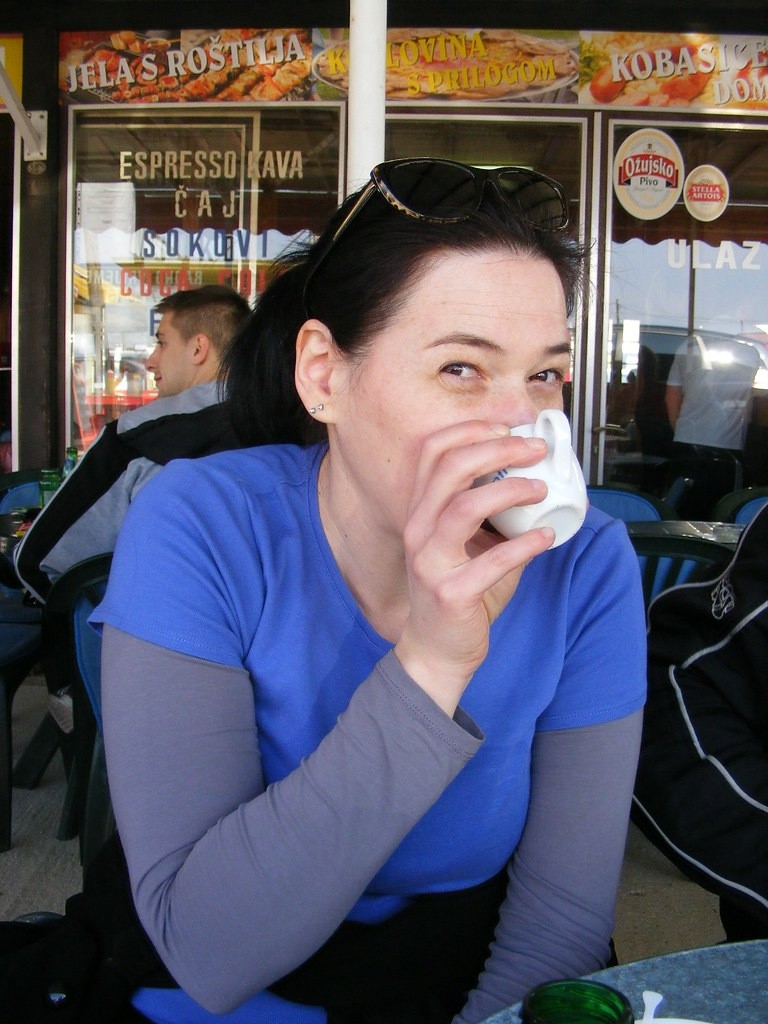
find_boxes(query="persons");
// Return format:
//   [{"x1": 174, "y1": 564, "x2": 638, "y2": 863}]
[
  {"x1": 664, "y1": 284, "x2": 768, "y2": 521},
  {"x1": 15, "y1": 285, "x2": 300, "y2": 733},
  {"x1": 88, "y1": 161, "x2": 649, "y2": 1024}
]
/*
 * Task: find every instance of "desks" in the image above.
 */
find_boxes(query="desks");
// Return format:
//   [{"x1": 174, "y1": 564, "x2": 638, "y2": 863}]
[{"x1": 0, "y1": 585, "x2": 41, "y2": 853}]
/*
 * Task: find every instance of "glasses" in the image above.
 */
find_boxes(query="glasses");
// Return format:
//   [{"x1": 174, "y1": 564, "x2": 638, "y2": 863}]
[{"x1": 302, "y1": 157, "x2": 570, "y2": 321}]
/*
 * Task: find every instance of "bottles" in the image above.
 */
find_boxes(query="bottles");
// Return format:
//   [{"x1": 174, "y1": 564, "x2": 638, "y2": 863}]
[
  {"x1": 106, "y1": 369, "x2": 115, "y2": 395},
  {"x1": 515, "y1": 979, "x2": 636, "y2": 1024},
  {"x1": 61, "y1": 447, "x2": 78, "y2": 481},
  {"x1": 38, "y1": 469, "x2": 61, "y2": 509}
]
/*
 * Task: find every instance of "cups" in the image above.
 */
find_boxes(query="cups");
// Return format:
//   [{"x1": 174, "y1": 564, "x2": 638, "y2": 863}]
[
  {"x1": 475, "y1": 409, "x2": 587, "y2": 550},
  {"x1": 9, "y1": 507, "x2": 27, "y2": 537}
]
